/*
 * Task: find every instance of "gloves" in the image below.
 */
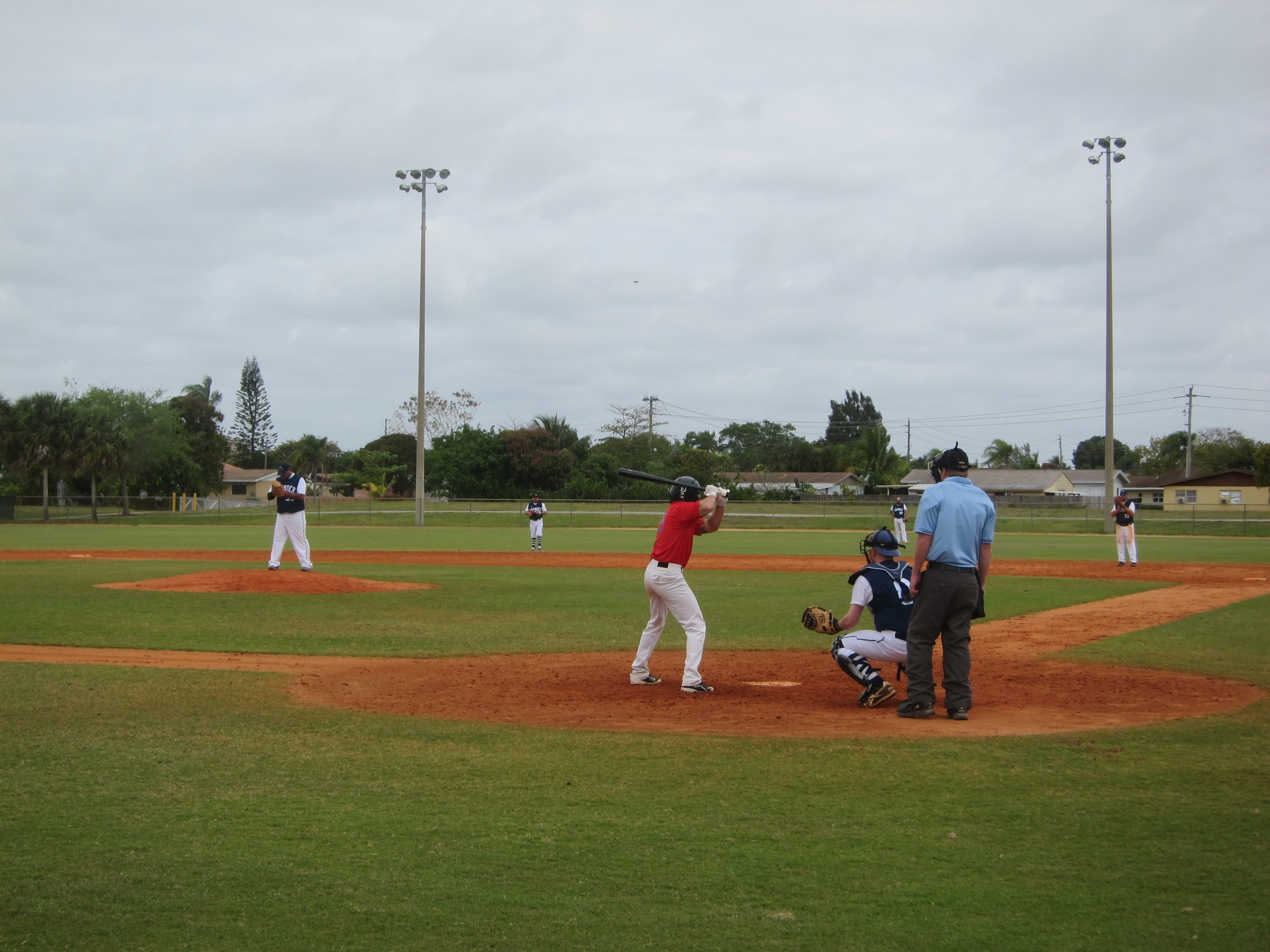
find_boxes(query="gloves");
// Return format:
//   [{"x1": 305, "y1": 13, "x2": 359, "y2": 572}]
[
  {"x1": 703, "y1": 485, "x2": 719, "y2": 499},
  {"x1": 716, "y1": 487, "x2": 730, "y2": 507}
]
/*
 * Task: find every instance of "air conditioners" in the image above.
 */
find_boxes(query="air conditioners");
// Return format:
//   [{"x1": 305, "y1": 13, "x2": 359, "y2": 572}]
[
  {"x1": 1178, "y1": 497, "x2": 1185, "y2": 503},
  {"x1": 1221, "y1": 499, "x2": 1227, "y2": 504}
]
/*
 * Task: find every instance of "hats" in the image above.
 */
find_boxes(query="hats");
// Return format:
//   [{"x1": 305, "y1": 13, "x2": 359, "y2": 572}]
[
  {"x1": 937, "y1": 446, "x2": 973, "y2": 472},
  {"x1": 532, "y1": 493, "x2": 539, "y2": 498},
  {"x1": 277, "y1": 462, "x2": 290, "y2": 476},
  {"x1": 896, "y1": 497, "x2": 901, "y2": 501},
  {"x1": 872, "y1": 529, "x2": 902, "y2": 558},
  {"x1": 1120, "y1": 489, "x2": 1128, "y2": 495}
]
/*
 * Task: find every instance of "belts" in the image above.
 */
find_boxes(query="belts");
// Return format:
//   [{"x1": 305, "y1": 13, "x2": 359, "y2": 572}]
[
  {"x1": 1117, "y1": 523, "x2": 1132, "y2": 526},
  {"x1": 928, "y1": 560, "x2": 977, "y2": 574},
  {"x1": 650, "y1": 558, "x2": 683, "y2": 571},
  {"x1": 531, "y1": 518, "x2": 541, "y2": 521},
  {"x1": 894, "y1": 517, "x2": 903, "y2": 519},
  {"x1": 896, "y1": 632, "x2": 906, "y2": 642}
]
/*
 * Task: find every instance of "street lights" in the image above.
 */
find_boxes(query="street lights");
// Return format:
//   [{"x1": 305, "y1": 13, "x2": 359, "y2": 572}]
[
  {"x1": 1082, "y1": 135, "x2": 1125, "y2": 536},
  {"x1": 396, "y1": 167, "x2": 451, "y2": 527}
]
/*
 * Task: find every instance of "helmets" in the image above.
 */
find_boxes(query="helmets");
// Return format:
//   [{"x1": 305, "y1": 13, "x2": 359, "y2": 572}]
[{"x1": 669, "y1": 476, "x2": 701, "y2": 503}]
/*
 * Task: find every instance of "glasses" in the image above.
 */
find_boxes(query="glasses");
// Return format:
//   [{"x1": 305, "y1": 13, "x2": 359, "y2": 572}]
[{"x1": 1120, "y1": 493, "x2": 1128, "y2": 496}]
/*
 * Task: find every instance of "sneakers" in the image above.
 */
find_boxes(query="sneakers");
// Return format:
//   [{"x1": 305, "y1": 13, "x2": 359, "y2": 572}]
[
  {"x1": 268, "y1": 565, "x2": 280, "y2": 570},
  {"x1": 947, "y1": 707, "x2": 969, "y2": 720},
  {"x1": 301, "y1": 567, "x2": 311, "y2": 571},
  {"x1": 530, "y1": 547, "x2": 536, "y2": 551},
  {"x1": 630, "y1": 672, "x2": 662, "y2": 686},
  {"x1": 858, "y1": 680, "x2": 897, "y2": 708},
  {"x1": 896, "y1": 698, "x2": 936, "y2": 717},
  {"x1": 537, "y1": 545, "x2": 542, "y2": 551},
  {"x1": 680, "y1": 680, "x2": 714, "y2": 693}
]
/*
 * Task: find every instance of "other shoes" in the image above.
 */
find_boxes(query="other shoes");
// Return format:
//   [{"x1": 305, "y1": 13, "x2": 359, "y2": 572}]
[
  {"x1": 1118, "y1": 561, "x2": 1125, "y2": 566},
  {"x1": 905, "y1": 541, "x2": 908, "y2": 543},
  {"x1": 1130, "y1": 562, "x2": 1137, "y2": 566}
]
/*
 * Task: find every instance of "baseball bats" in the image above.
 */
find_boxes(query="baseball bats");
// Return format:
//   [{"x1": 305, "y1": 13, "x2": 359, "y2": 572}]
[{"x1": 618, "y1": 467, "x2": 730, "y2": 499}]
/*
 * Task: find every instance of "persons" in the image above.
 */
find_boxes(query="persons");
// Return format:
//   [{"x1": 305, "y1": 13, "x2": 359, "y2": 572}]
[
  {"x1": 525, "y1": 493, "x2": 547, "y2": 551},
  {"x1": 801, "y1": 527, "x2": 920, "y2": 707},
  {"x1": 897, "y1": 441, "x2": 995, "y2": 719},
  {"x1": 890, "y1": 497, "x2": 908, "y2": 544},
  {"x1": 630, "y1": 476, "x2": 729, "y2": 692},
  {"x1": 267, "y1": 463, "x2": 313, "y2": 572},
  {"x1": 1111, "y1": 489, "x2": 1137, "y2": 566}
]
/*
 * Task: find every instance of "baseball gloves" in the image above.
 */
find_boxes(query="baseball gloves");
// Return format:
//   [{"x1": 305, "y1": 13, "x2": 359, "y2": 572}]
[
  {"x1": 530, "y1": 510, "x2": 541, "y2": 519},
  {"x1": 1114, "y1": 495, "x2": 1126, "y2": 508},
  {"x1": 904, "y1": 519, "x2": 908, "y2": 522},
  {"x1": 800, "y1": 605, "x2": 844, "y2": 635}
]
